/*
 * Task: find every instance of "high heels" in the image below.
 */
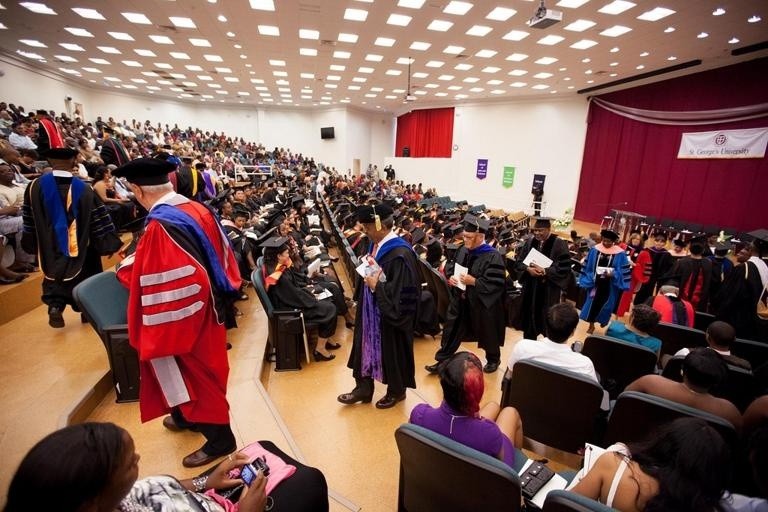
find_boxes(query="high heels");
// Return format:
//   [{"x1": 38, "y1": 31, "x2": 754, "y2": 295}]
[
  {"x1": 326, "y1": 342, "x2": 340, "y2": 350},
  {"x1": 330, "y1": 258, "x2": 339, "y2": 262},
  {"x1": 346, "y1": 322, "x2": 355, "y2": 331},
  {"x1": 313, "y1": 351, "x2": 335, "y2": 362}
]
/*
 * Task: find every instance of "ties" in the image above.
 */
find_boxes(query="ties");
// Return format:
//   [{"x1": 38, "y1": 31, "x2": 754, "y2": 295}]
[{"x1": 372, "y1": 242, "x2": 378, "y2": 257}]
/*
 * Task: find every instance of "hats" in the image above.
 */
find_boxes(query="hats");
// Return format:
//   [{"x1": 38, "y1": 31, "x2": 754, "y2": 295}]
[
  {"x1": 43, "y1": 149, "x2": 78, "y2": 160},
  {"x1": 331, "y1": 191, "x2": 528, "y2": 249},
  {"x1": 37, "y1": 110, "x2": 47, "y2": 115},
  {"x1": 630, "y1": 229, "x2": 731, "y2": 251},
  {"x1": 601, "y1": 230, "x2": 619, "y2": 242},
  {"x1": 209, "y1": 179, "x2": 305, "y2": 253},
  {"x1": 164, "y1": 145, "x2": 206, "y2": 169},
  {"x1": 111, "y1": 158, "x2": 178, "y2": 185},
  {"x1": 104, "y1": 128, "x2": 114, "y2": 134},
  {"x1": 746, "y1": 229, "x2": 768, "y2": 242},
  {"x1": 534, "y1": 217, "x2": 556, "y2": 228}
]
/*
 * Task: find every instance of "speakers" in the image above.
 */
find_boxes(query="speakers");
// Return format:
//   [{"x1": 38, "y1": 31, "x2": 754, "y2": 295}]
[{"x1": 321, "y1": 127, "x2": 334, "y2": 139}]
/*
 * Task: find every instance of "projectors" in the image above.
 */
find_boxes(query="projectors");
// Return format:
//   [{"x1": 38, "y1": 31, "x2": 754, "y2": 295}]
[
  {"x1": 406, "y1": 96, "x2": 418, "y2": 101},
  {"x1": 529, "y1": 9, "x2": 563, "y2": 29}
]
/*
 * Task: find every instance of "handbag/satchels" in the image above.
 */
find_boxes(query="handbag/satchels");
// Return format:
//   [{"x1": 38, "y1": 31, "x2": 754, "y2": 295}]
[
  {"x1": 757, "y1": 297, "x2": 768, "y2": 320},
  {"x1": 519, "y1": 458, "x2": 555, "y2": 499},
  {"x1": 227, "y1": 442, "x2": 295, "y2": 497}
]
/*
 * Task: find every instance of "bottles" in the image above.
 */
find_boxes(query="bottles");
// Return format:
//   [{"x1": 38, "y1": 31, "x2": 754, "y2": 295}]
[
  {"x1": 365, "y1": 264, "x2": 376, "y2": 293},
  {"x1": 602, "y1": 269, "x2": 610, "y2": 275}
]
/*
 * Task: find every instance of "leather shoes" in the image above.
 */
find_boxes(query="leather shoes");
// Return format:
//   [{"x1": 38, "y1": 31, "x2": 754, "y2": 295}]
[
  {"x1": 338, "y1": 393, "x2": 372, "y2": 404},
  {"x1": 163, "y1": 416, "x2": 178, "y2": 430},
  {"x1": 483, "y1": 360, "x2": 500, "y2": 373},
  {"x1": 81, "y1": 313, "x2": 87, "y2": 323},
  {"x1": 425, "y1": 359, "x2": 449, "y2": 373},
  {"x1": 376, "y1": 393, "x2": 406, "y2": 409},
  {"x1": 0, "y1": 262, "x2": 36, "y2": 284},
  {"x1": 49, "y1": 307, "x2": 64, "y2": 328},
  {"x1": 183, "y1": 445, "x2": 237, "y2": 467}
]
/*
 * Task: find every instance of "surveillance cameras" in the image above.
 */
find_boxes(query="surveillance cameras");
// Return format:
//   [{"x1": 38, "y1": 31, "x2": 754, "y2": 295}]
[{"x1": 64, "y1": 94, "x2": 72, "y2": 101}]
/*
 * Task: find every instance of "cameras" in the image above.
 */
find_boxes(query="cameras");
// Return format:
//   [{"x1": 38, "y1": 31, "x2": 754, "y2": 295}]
[{"x1": 240, "y1": 457, "x2": 270, "y2": 488}]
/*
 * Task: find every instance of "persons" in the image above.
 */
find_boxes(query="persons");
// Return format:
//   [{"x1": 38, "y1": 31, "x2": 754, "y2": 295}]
[
  {"x1": 1, "y1": 101, "x2": 425, "y2": 511},
  {"x1": 427, "y1": 187, "x2": 766, "y2": 511}
]
